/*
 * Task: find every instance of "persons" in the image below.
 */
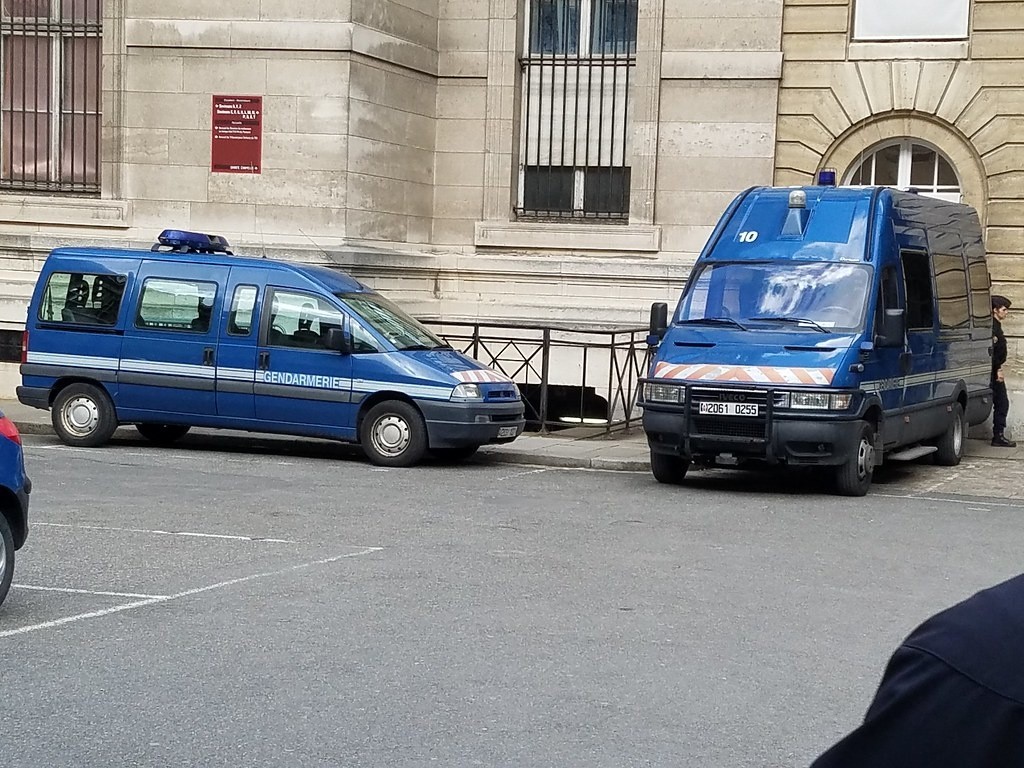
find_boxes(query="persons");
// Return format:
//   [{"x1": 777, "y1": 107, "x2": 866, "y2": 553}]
[
  {"x1": 990, "y1": 295, "x2": 1016, "y2": 447},
  {"x1": 808, "y1": 572, "x2": 1024, "y2": 768}
]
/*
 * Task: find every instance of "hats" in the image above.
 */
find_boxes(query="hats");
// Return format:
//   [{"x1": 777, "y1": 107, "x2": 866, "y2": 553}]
[{"x1": 992, "y1": 295, "x2": 1011, "y2": 309}]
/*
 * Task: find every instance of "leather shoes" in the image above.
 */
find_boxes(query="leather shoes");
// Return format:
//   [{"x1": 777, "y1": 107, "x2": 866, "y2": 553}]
[{"x1": 991, "y1": 436, "x2": 1016, "y2": 446}]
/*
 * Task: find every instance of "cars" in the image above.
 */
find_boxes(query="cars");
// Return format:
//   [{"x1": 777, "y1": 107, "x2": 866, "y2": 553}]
[{"x1": 15, "y1": 228, "x2": 528, "y2": 466}]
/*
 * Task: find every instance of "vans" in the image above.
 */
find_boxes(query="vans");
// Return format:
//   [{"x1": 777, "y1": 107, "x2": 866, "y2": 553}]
[{"x1": 636, "y1": 168, "x2": 995, "y2": 498}]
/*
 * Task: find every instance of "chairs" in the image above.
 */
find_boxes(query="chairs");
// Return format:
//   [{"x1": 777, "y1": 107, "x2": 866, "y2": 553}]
[
  {"x1": 294, "y1": 303, "x2": 323, "y2": 345},
  {"x1": 191, "y1": 292, "x2": 214, "y2": 331},
  {"x1": 271, "y1": 296, "x2": 281, "y2": 335},
  {"x1": 61, "y1": 279, "x2": 98, "y2": 323}
]
